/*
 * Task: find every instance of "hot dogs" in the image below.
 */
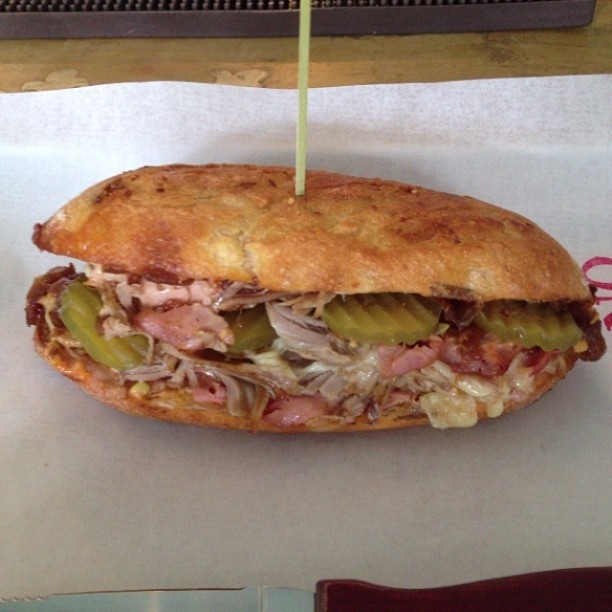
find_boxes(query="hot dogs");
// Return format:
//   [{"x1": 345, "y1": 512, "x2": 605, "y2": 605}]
[{"x1": 24, "y1": 163, "x2": 607, "y2": 433}]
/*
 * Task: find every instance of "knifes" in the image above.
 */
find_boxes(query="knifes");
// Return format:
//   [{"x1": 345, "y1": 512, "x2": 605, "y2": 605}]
[{"x1": 0, "y1": 566, "x2": 611, "y2": 612}]
